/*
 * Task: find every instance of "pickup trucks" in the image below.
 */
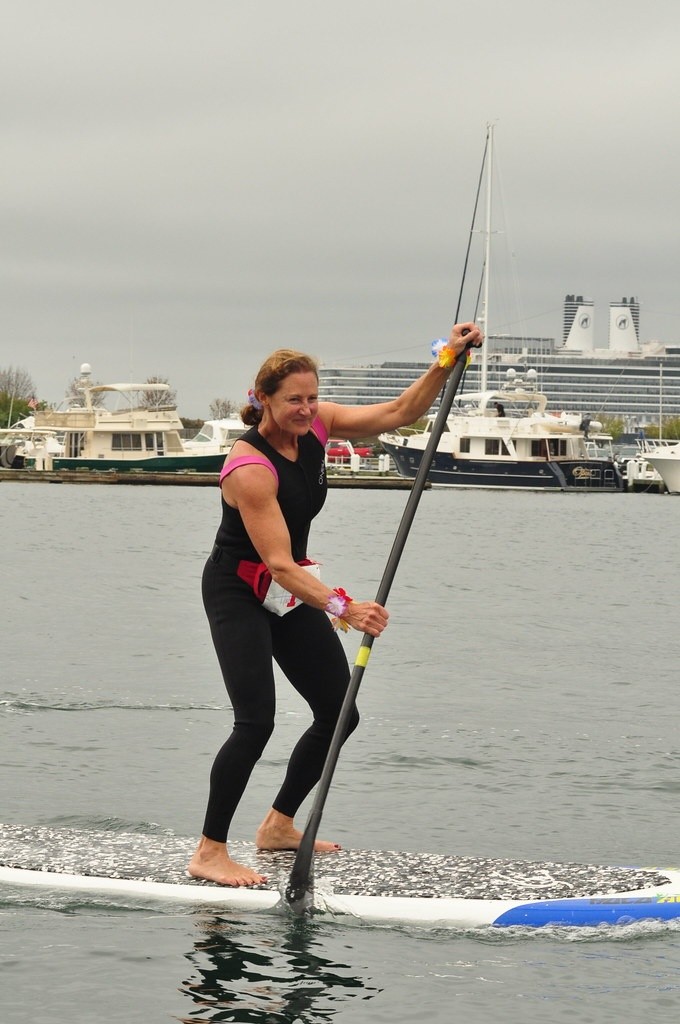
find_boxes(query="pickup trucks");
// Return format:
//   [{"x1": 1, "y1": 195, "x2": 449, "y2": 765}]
[{"x1": 325, "y1": 439, "x2": 372, "y2": 462}]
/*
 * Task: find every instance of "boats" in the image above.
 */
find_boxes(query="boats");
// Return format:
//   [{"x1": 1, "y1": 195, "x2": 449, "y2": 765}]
[
  {"x1": 635, "y1": 363, "x2": 680, "y2": 494},
  {"x1": 621, "y1": 459, "x2": 666, "y2": 492},
  {"x1": 1, "y1": 364, "x2": 255, "y2": 470}
]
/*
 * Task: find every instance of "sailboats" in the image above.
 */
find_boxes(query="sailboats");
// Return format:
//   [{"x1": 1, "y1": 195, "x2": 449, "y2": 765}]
[{"x1": 377, "y1": 122, "x2": 626, "y2": 491}]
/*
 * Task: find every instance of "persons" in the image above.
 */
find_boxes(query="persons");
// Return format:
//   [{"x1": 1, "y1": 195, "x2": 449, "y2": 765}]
[{"x1": 184, "y1": 317, "x2": 488, "y2": 889}]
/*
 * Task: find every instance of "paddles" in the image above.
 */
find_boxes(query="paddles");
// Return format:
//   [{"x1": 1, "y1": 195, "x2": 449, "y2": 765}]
[{"x1": 285, "y1": 328, "x2": 486, "y2": 903}]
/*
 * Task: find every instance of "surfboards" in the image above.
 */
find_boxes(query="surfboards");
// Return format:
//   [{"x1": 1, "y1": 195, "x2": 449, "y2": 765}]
[{"x1": 1, "y1": 824, "x2": 680, "y2": 935}]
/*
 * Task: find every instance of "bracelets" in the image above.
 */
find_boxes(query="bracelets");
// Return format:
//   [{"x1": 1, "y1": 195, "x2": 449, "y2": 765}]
[
  {"x1": 325, "y1": 585, "x2": 357, "y2": 636},
  {"x1": 429, "y1": 337, "x2": 476, "y2": 370}
]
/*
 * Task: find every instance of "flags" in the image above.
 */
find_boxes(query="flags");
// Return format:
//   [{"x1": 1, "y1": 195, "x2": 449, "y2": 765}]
[{"x1": 27, "y1": 397, "x2": 39, "y2": 410}]
